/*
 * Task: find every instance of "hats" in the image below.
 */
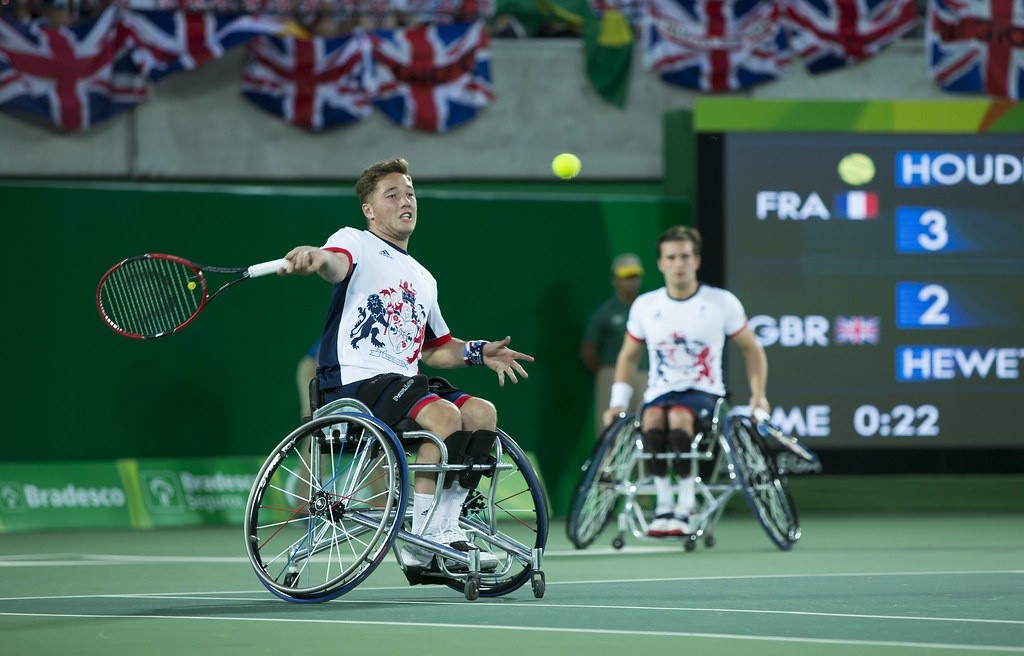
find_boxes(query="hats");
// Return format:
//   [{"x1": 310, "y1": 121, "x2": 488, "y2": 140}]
[{"x1": 610, "y1": 253, "x2": 643, "y2": 279}]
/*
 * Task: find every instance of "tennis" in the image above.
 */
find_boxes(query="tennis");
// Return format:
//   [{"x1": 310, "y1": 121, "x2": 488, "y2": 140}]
[{"x1": 550, "y1": 151, "x2": 582, "y2": 181}]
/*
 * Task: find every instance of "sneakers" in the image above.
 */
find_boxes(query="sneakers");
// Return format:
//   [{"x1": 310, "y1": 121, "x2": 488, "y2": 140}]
[{"x1": 401, "y1": 518, "x2": 499, "y2": 573}]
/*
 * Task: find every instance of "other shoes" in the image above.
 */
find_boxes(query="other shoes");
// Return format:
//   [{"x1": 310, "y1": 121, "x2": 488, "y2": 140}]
[
  {"x1": 647, "y1": 506, "x2": 674, "y2": 536},
  {"x1": 666, "y1": 505, "x2": 697, "y2": 535}
]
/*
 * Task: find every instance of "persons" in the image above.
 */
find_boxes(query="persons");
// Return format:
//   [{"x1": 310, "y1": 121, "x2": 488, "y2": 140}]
[
  {"x1": 579, "y1": 222, "x2": 772, "y2": 540},
  {"x1": 274, "y1": 158, "x2": 535, "y2": 575}
]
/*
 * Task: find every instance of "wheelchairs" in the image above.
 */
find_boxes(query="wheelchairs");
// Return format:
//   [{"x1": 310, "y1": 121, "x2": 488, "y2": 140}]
[
  {"x1": 566, "y1": 396, "x2": 802, "y2": 552},
  {"x1": 242, "y1": 376, "x2": 550, "y2": 606}
]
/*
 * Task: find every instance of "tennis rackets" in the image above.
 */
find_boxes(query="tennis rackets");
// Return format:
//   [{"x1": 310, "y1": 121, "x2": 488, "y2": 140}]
[
  {"x1": 751, "y1": 406, "x2": 815, "y2": 462},
  {"x1": 94, "y1": 251, "x2": 291, "y2": 342}
]
